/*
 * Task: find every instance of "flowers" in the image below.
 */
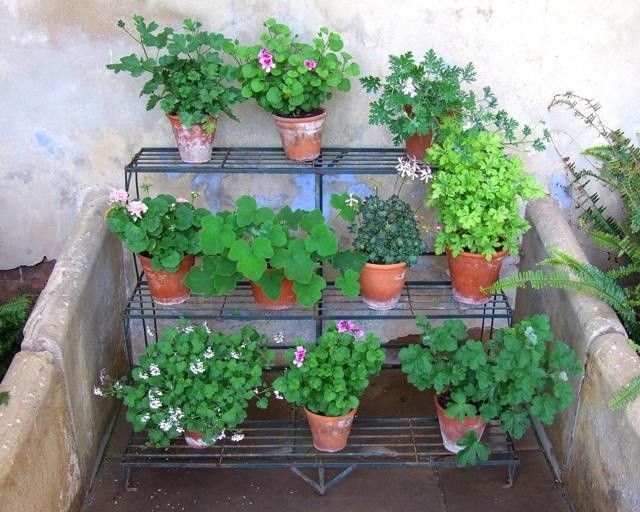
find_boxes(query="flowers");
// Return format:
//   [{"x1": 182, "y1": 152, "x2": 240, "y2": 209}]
[{"x1": 236, "y1": 18, "x2": 361, "y2": 108}]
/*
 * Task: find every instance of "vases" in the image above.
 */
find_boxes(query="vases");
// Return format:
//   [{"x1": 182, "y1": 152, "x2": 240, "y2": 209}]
[{"x1": 271, "y1": 109, "x2": 327, "y2": 162}]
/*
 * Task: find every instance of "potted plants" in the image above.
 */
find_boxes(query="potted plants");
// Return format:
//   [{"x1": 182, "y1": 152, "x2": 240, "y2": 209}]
[
  {"x1": 103, "y1": 14, "x2": 237, "y2": 164},
  {"x1": 345, "y1": 157, "x2": 438, "y2": 310},
  {"x1": 95, "y1": 314, "x2": 284, "y2": 449},
  {"x1": 422, "y1": 131, "x2": 545, "y2": 306},
  {"x1": 271, "y1": 320, "x2": 387, "y2": 454},
  {"x1": 180, "y1": 195, "x2": 341, "y2": 310},
  {"x1": 399, "y1": 312, "x2": 577, "y2": 468},
  {"x1": 358, "y1": 50, "x2": 551, "y2": 159},
  {"x1": 104, "y1": 181, "x2": 212, "y2": 306}
]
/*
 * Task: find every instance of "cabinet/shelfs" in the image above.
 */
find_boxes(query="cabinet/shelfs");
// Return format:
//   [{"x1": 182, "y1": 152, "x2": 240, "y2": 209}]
[{"x1": 115, "y1": 147, "x2": 523, "y2": 493}]
